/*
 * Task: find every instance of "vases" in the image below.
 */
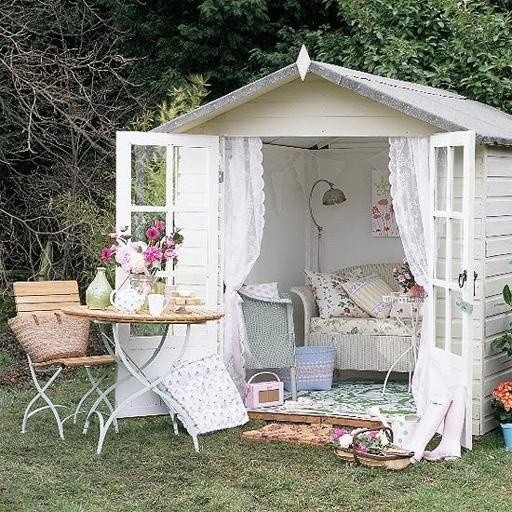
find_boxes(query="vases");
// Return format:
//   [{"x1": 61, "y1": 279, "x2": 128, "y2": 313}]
[
  {"x1": 85, "y1": 267, "x2": 114, "y2": 309},
  {"x1": 128, "y1": 276, "x2": 156, "y2": 313},
  {"x1": 500, "y1": 423, "x2": 512, "y2": 450}
]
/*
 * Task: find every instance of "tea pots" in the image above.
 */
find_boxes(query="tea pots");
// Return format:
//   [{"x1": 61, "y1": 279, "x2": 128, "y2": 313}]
[{"x1": 110, "y1": 289, "x2": 146, "y2": 314}]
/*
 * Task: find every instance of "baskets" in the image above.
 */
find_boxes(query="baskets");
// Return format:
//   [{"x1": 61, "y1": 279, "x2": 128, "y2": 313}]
[
  {"x1": 278, "y1": 337, "x2": 337, "y2": 392},
  {"x1": 333, "y1": 427, "x2": 415, "y2": 471}
]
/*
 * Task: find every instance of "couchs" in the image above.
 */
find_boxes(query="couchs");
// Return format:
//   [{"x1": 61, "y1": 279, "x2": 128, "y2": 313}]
[{"x1": 288, "y1": 262, "x2": 422, "y2": 383}]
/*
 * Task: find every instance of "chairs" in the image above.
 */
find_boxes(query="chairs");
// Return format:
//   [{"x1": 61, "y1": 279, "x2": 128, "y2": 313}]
[
  {"x1": 13, "y1": 280, "x2": 130, "y2": 440},
  {"x1": 236, "y1": 283, "x2": 297, "y2": 401}
]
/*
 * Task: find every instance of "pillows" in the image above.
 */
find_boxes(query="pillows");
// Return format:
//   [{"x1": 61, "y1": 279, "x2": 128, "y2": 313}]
[
  {"x1": 240, "y1": 282, "x2": 280, "y2": 300},
  {"x1": 304, "y1": 268, "x2": 370, "y2": 318},
  {"x1": 341, "y1": 272, "x2": 399, "y2": 318}
]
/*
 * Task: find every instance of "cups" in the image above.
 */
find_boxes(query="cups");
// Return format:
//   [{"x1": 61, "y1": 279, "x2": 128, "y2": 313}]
[{"x1": 147, "y1": 293, "x2": 169, "y2": 316}]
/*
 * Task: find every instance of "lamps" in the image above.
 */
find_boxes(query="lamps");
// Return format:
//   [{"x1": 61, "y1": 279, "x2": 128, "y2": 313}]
[{"x1": 309, "y1": 178, "x2": 347, "y2": 273}]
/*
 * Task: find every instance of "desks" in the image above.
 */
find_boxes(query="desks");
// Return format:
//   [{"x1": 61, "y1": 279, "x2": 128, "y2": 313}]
[{"x1": 61, "y1": 306, "x2": 226, "y2": 454}]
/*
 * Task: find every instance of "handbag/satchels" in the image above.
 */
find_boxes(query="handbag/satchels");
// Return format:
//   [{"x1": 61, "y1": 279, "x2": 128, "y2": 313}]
[{"x1": 8, "y1": 313, "x2": 90, "y2": 362}]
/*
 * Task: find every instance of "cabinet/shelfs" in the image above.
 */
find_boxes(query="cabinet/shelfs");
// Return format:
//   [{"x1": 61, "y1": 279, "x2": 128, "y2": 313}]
[{"x1": 383, "y1": 293, "x2": 430, "y2": 396}]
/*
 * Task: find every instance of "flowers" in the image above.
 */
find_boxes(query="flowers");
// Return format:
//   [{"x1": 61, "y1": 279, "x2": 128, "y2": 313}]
[
  {"x1": 392, "y1": 254, "x2": 416, "y2": 294},
  {"x1": 491, "y1": 379, "x2": 512, "y2": 423},
  {"x1": 324, "y1": 425, "x2": 414, "y2": 459},
  {"x1": 100, "y1": 219, "x2": 184, "y2": 276}
]
